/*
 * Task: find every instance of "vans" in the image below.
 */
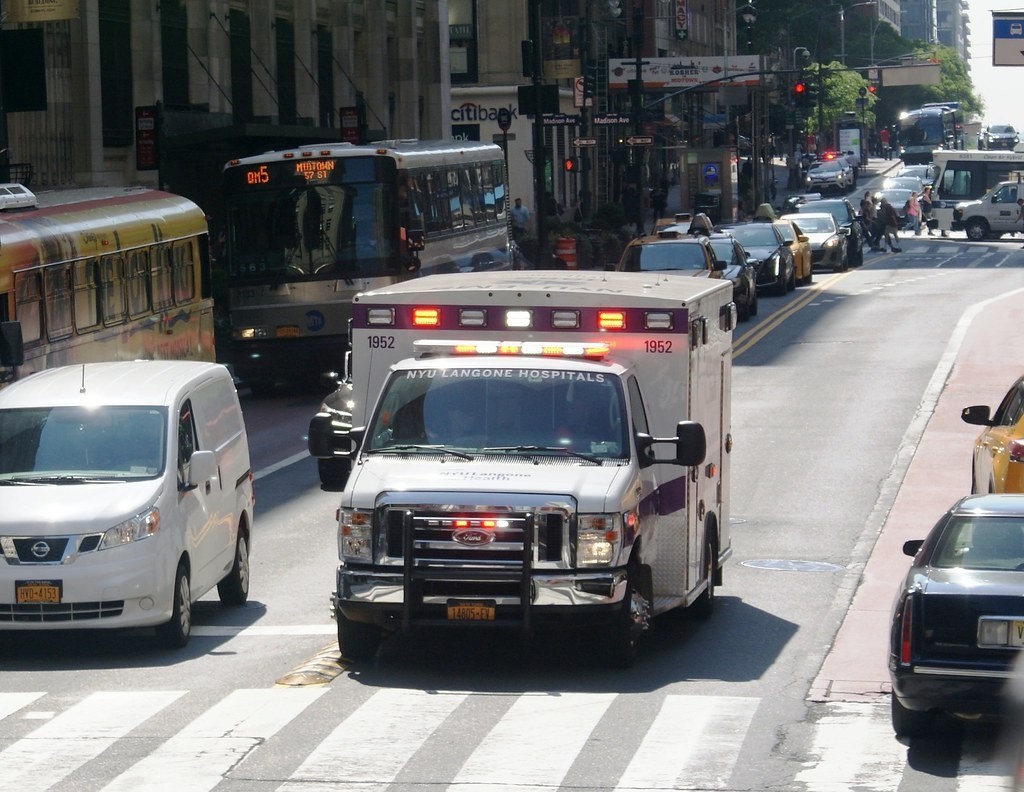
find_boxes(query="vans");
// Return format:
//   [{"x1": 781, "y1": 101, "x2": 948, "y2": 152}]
[{"x1": 1, "y1": 357, "x2": 256, "y2": 651}]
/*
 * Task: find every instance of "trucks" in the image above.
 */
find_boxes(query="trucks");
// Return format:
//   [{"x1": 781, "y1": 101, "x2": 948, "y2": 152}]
[{"x1": 950, "y1": 171, "x2": 1024, "y2": 242}]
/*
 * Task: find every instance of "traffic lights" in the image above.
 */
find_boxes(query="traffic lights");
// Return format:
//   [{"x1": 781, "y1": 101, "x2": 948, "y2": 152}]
[
  {"x1": 794, "y1": 80, "x2": 806, "y2": 105},
  {"x1": 566, "y1": 157, "x2": 579, "y2": 173},
  {"x1": 805, "y1": 80, "x2": 818, "y2": 107}
]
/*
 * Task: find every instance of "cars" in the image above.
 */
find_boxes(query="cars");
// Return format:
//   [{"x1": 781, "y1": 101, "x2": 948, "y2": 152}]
[
  {"x1": 961, "y1": 375, "x2": 1024, "y2": 496},
  {"x1": 976, "y1": 128, "x2": 988, "y2": 150},
  {"x1": 983, "y1": 123, "x2": 1020, "y2": 151},
  {"x1": 603, "y1": 150, "x2": 936, "y2": 324},
  {"x1": 888, "y1": 492, "x2": 1024, "y2": 742},
  {"x1": 305, "y1": 350, "x2": 362, "y2": 493}
]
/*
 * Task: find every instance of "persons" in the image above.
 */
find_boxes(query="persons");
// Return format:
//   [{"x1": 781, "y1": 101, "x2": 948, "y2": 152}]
[
  {"x1": 858, "y1": 192, "x2": 904, "y2": 250},
  {"x1": 1015, "y1": 199, "x2": 1024, "y2": 248},
  {"x1": 760, "y1": 140, "x2": 784, "y2": 161},
  {"x1": 575, "y1": 196, "x2": 588, "y2": 227},
  {"x1": 902, "y1": 191, "x2": 922, "y2": 236},
  {"x1": 870, "y1": 126, "x2": 889, "y2": 157},
  {"x1": 618, "y1": 170, "x2": 675, "y2": 226},
  {"x1": 797, "y1": 131, "x2": 815, "y2": 155},
  {"x1": 510, "y1": 198, "x2": 530, "y2": 240},
  {"x1": 742, "y1": 156, "x2": 753, "y2": 181},
  {"x1": 921, "y1": 187, "x2": 935, "y2": 236},
  {"x1": 540, "y1": 193, "x2": 558, "y2": 218},
  {"x1": 999, "y1": 189, "x2": 1016, "y2": 201}
]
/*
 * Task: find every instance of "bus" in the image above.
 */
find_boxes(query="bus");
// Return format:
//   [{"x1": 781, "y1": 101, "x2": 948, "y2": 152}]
[
  {"x1": 1, "y1": 183, "x2": 218, "y2": 398},
  {"x1": 926, "y1": 142, "x2": 1024, "y2": 240},
  {"x1": 896, "y1": 101, "x2": 967, "y2": 166},
  {"x1": 209, "y1": 138, "x2": 517, "y2": 387}
]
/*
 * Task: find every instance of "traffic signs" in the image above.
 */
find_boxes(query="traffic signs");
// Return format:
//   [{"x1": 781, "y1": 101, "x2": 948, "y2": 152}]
[
  {"x1": 570, "y1": 137, "x2": 598, "y2": 148},
  {"x1": 624, "y1": 136, "x2": 655, "y2": 147}
]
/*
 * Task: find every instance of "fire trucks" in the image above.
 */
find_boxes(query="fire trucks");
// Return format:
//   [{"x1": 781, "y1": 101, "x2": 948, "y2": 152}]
[{"x1": 307, "y1": 267, "x2": 733, "y2": 672}]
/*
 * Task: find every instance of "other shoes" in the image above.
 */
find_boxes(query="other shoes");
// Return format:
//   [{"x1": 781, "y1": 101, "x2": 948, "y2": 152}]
[
  {"x1": 928, "y1": 232, "x2": 935, "y2": 235},
  {"x1": 902, "y1": 227, "x2": 906, "y2": 233},
  {"x1": 880, "y1": 248, "x2": 887, "y2": 252},
  {"x1": 891, "y1": 247, "x2": 902, "y2": 252}
]
